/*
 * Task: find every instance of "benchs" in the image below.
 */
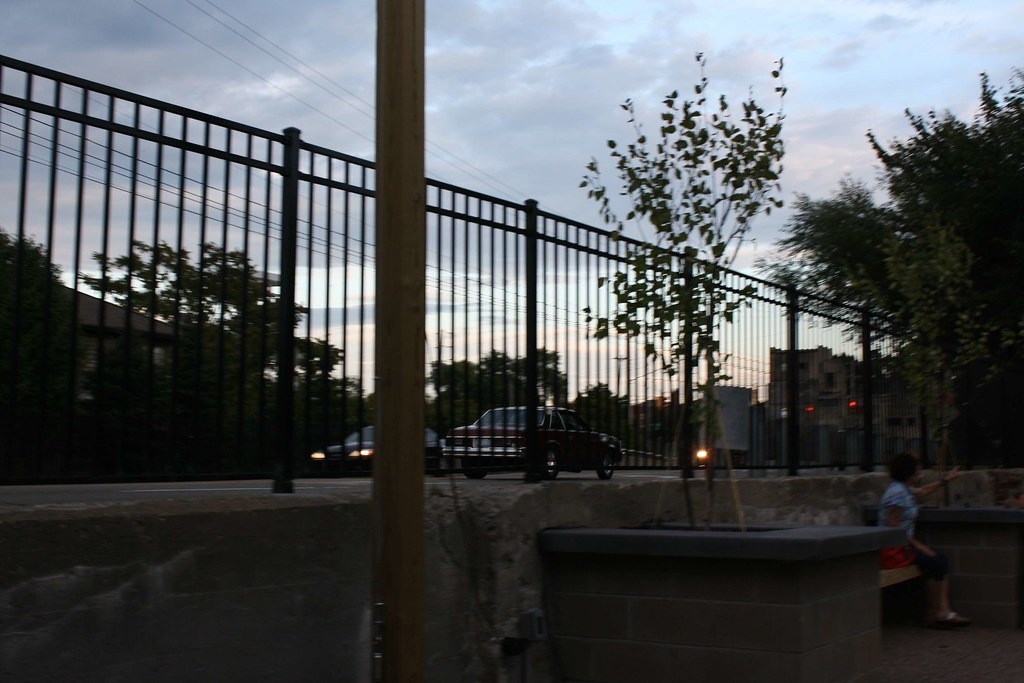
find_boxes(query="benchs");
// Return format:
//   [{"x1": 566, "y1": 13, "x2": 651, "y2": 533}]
[{"x1": 877, "y1": 559, "x2": 921, "y2": 586}]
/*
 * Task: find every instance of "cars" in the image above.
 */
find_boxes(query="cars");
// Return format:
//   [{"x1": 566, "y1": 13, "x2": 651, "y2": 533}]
[
  {"x1": 311, "y1": 423, "x2": 449, "y2": 478},
  {"x1": 443, "y1": 408, "x2": 621, "y2": 481}
]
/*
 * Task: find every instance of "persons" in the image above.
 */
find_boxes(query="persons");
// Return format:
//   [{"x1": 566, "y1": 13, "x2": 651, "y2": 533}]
[
  {"x1": 877, "y1": 452, "x2": 970, "y2": 628},
  {"x1": 424, "y1": 421, "x2": 437, "y2": 474}
]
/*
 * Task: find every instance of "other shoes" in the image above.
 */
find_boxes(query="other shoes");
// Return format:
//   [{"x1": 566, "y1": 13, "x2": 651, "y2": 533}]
[{"x1": 921, "y1": 612, "x2": 971, "y2": 627}]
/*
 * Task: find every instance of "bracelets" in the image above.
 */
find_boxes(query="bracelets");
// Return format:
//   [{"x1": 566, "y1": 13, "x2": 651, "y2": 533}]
[{"x1": 941, "y1": 478, "x2": 948, "y2": 485}]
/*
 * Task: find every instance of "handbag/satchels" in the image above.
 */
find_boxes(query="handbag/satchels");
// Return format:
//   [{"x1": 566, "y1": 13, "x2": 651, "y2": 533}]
[{"x1": 880, "y1": 546, "x2": 915, "y2": 568}]
